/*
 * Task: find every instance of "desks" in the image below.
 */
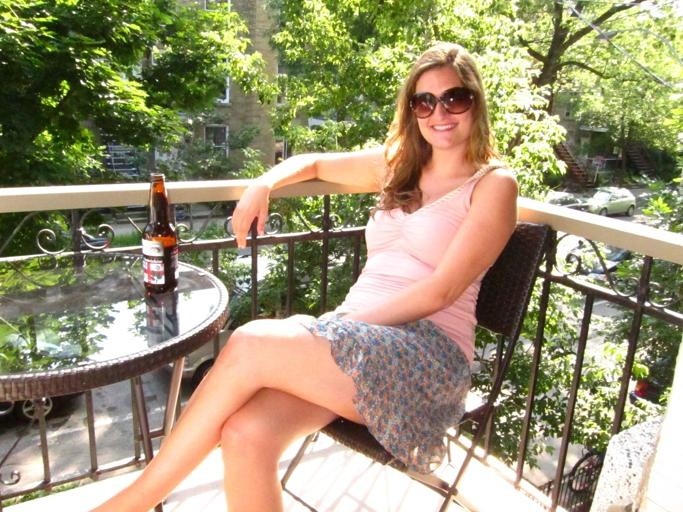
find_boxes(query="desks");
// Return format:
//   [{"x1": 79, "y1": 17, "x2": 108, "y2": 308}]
[{"x1": 1, "y1": 250, "x2": 229, "y2": 512}]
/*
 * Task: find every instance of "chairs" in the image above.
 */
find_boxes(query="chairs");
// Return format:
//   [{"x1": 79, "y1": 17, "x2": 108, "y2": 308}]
[{"x1": 279, "y1": 223, "x2": 681, "y2": 511}]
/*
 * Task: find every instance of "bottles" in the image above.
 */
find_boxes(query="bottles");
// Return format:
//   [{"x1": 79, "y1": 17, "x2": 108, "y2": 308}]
[{"x1": 141, "y1": 172, "x2": 180, "y2": 294}]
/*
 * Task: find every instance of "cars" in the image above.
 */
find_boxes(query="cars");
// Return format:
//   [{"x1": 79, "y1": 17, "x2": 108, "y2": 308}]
[
  {"x1": 458, "y1": 372, "x2": 533, "y2": 441},
  {"x1": 0, "y1": 392, "x2": 88, "y2": 424},
  {"x1": 541, "y1": 184, "x2": 638, "y2": 287},
  {"x1": 60, "y1": 233, "x2": 114, "y2": 252},
  {"x1": 160, "y1": 247, "x2": 355, "y2": 389},
  {"x1": 628, "y1": 349, "x2": 671, "y2": 414}
]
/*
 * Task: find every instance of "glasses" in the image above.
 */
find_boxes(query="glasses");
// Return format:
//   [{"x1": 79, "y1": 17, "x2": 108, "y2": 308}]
[{"x1": 410, "y1": 86, "x2": 475, "y2": 118}]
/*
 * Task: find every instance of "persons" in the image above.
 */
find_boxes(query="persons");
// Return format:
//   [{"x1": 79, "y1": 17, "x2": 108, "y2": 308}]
[{"x1": 87, "y1": 41, "x2": 519, "y2": 512}]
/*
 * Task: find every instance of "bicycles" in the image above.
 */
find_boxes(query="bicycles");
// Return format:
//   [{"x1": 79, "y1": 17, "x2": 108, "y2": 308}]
[{"x1": 567, "y1": 429, "x2": 606, "y2": 493}]
